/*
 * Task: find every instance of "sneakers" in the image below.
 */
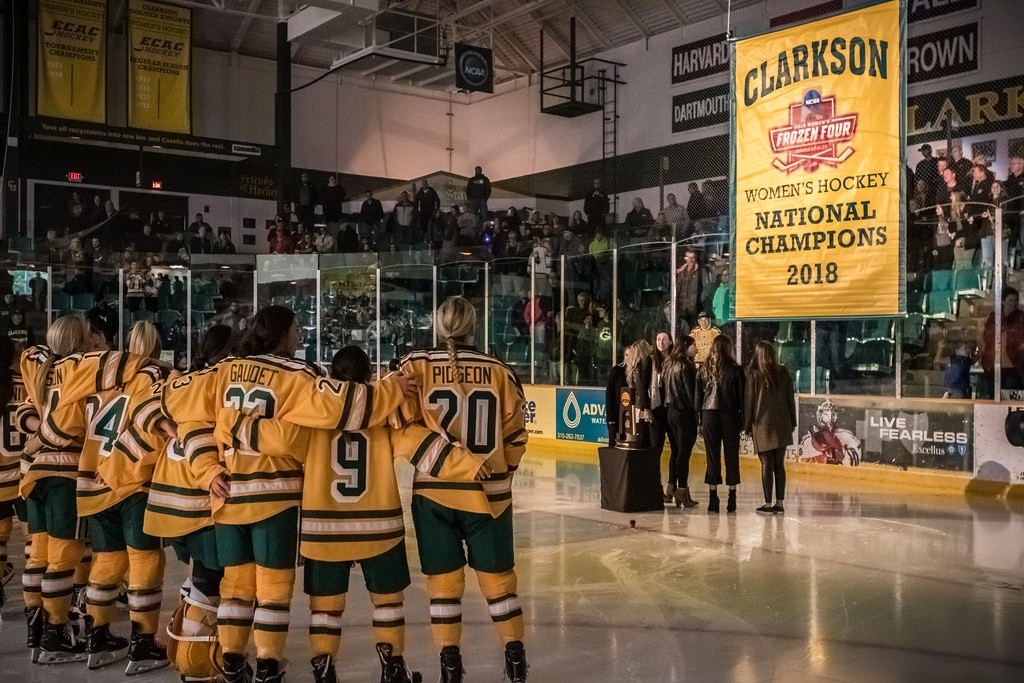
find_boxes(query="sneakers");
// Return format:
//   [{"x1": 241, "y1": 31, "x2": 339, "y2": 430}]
[
  {"x1": 223, "y1": 652, "x2": 254, "y2": 683},
  {"x1": 115, "y1": 588, "x2": 130, "y2": 609},
  {"x1": 24, "y1": 583, "x2": 88, "y2": 664},
  {"x1": 83, "y1": 615, "x2": 131, "y2": 668},
  {"x1": 505, "y1": 641, "x2": 527, "y2": 683},
  {"x1": 376, "y1": 643, "x2": 422, "y2": 683},
  {"x1": 311, "y1": 654, "x2": 337, "y2": 683},
  {"x1": 124, "y1": 620, "x2": 172, "y2": 675},
  {"x1": 255, "y1": 657, "x2": 291, "y2": 683},
  {"x1": 439, "y1": 646, "x2": 463, "y2": 683},
  {"x1": 0, "y1": 563, "x2": 15, "y2": 608}
]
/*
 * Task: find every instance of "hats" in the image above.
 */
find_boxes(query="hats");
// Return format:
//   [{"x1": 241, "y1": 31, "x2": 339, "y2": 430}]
[
  {"x1": 918, "y1": 144, "x2": 931, "y2": 151},
  {"x1": 697, "y1": 311, "x2": 712, "y2": 320},
  {"x1": 596, "y1": 304, "x2": 609, "y2": 312},
  {"x1": 89, "y1": 307, "x2": 119, "y2": 341}
]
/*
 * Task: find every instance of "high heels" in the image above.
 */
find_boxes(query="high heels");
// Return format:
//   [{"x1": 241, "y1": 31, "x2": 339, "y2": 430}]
[
  {"x1": 665, "y1": 482, "x2": 678, "y2": 500},
  {"x1": 727, "y1": 489, "x2": 737, "y2": 512},
  {"x1": 675, "y1": 487, "x2": 699, "y2": 508},
  {"x1": 708, "y1": 490, "x2": 719, "y2": 513}
]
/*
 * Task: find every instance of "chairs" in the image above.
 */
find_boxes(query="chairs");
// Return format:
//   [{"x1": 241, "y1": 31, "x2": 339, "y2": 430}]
[{"x1": 3, "y1": 233, "x2": 992, "y2": 395}]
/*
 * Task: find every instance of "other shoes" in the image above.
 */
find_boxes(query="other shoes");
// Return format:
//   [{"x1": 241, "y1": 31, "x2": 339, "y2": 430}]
[
  {"x1": 757, "y1": 505, "x2": 773, "y2": 515},
  {"x1": 772, "y1": 504, "x2": 785, "y2": 514}
]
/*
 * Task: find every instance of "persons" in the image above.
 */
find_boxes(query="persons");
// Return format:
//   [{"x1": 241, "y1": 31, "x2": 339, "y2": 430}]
[
  {"x1": 28, "y1": 190, "x2": 236, "y2": 305},
  {"x1": 980, "y1": 286, "x2": 1024, "y2": 385},
  {"x1": 512, "y1": 288, "x2": 620, "y2": 387},
  {"x1": 942, "y1": 342, "x2": 974, "y2": 399},
  {"x1": 798, "y1": 401, "x2": 861, "y2": 466},
  {"x1": 266, "y1": 164, "x2": 730, "y2": 294},
  {"x1": 604, "y1": 250, "x2": 855, "y2": 515},
  {"x1": 0, "y1": 294, "x2": 531, "y2": 683},
  {"x1": 906, "y1": 144, "x2": 1024, "y2": 276}
]
